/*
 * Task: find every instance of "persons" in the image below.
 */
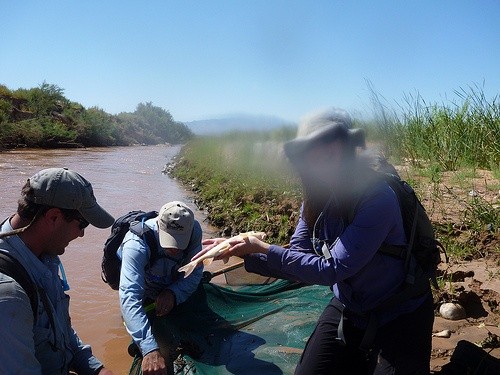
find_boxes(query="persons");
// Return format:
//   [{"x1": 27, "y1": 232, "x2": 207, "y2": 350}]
[
  {"x1": 118, "y1": 201, "x2": 204, "y2": 375},
  {"x1": 189, "y1": 106, "x2": 435, "y2": 375},
  {"x1": 0, "y1": 167, "x2": 114, "y2": 375}
]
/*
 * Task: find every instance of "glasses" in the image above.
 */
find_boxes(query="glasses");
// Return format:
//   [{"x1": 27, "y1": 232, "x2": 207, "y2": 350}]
[{"x1": 68, "y1": 214, "x2": 90, "y2": 230}]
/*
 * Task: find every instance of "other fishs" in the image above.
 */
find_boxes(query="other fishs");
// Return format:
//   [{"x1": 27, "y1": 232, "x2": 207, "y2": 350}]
[{"x1": 178, "y1": 230, "x2": 266, "y2": 278}]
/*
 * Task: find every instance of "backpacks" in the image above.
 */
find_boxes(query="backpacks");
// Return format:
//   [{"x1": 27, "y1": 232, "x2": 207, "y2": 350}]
[
  {"x1": 343, "y1": 162, "x2": 442, "y2": 279},
  {"x1": 101, "y1": 210, "x2": 159, "y2": 291}
]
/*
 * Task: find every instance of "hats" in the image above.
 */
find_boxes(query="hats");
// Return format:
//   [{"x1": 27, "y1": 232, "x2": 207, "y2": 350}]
[
  {"x1": 282, "y1": 107, "x2": 367, "y2": 162},
  {"x1": 158, "y1": 201, "x2": 194, "y2": 250},
  {"x1": 20, "y1": 167, "x2": 114, "y2": 229}
]
